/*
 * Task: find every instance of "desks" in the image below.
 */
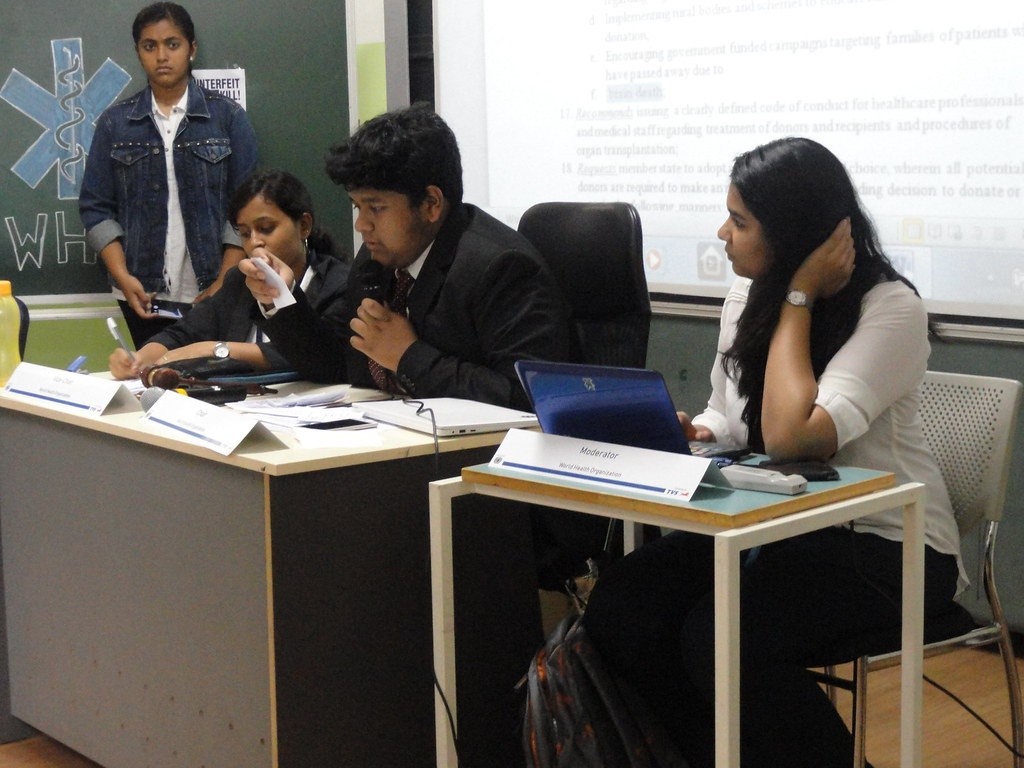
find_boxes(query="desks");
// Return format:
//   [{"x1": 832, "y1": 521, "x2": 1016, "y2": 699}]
[
  {"x1": 428, "y1": 449, "x2": 926, "y2": 768},
  {"x1": 0, "y1": 372, "x2": 541, "y2": 768}
]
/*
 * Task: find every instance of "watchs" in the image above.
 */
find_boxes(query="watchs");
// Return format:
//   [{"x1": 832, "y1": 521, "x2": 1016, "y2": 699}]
[
  {"x1": 783, "y1": 289, "x2": 815, "y2": 312},
  {"x1": 213, "y1": 340, "x2": 231, "y2": 358}
]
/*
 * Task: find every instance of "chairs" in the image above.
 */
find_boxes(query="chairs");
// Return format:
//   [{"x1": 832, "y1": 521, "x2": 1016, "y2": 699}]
[
  {"x1": 517, "y1": 203, "x2": 651, "y2": 371},
  {"x1": 804, "y1": 370, "x2": 1024, "y2": 768}
]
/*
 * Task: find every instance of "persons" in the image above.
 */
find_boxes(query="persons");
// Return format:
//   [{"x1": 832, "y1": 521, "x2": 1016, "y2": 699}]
[
  {"x1": 79, "y1": 0, "x2": 263, "y2": 351},
  {"x1": 238, "y1": 102, "x2": 584, "y2": 414},
  {"x1": 582, "y1": 137, "x2": 971, "y2": 768},
  {"x1": 107, "y1": 169, "x2": 355, "y2": 384}
]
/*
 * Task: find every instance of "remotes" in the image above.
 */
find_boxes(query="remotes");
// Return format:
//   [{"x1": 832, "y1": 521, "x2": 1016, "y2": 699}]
[{"x1": 721, "y1": 463, "x2": 807, "y2": 494}]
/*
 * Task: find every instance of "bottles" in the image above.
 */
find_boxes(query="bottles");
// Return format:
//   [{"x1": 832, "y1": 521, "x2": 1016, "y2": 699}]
[{"x1": 0, "y1": 279, "x2": 22, "y2": 388}]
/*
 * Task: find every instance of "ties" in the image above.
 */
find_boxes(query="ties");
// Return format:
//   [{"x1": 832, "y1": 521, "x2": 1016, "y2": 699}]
[{"x1": 368, "y1": 267, "x2": 416, "y2": 390}]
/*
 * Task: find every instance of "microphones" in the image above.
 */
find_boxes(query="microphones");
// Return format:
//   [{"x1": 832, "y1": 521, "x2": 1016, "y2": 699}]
[
  {"x1": 354, "y1": 259, "x2": 384, "y2": 310},
  {"x1": 139, "y1": 386, "x2": 248, "y2": 413}
]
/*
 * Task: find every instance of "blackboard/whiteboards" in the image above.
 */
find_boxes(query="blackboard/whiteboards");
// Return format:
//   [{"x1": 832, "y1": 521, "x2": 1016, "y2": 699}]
[{"x1": 0, "y1": 0, "x2": 361, "y2": 323}]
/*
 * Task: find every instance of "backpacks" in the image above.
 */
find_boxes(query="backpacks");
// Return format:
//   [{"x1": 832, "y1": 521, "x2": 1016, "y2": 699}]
[{"x1": 514, "y1": 613, "x2": 688, "y2": 768}]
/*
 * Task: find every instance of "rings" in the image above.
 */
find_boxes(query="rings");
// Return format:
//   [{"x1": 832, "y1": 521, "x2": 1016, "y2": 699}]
[{"x1": 163, "y1": 356, "x2": 168, "y2": 360}]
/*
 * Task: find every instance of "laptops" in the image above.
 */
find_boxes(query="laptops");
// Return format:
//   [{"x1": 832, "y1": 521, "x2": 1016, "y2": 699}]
[
  {"x1": 353, "y1": 397, "x2": 541, "y2": 436},
  {"x1": 513, "y1": 359, "x2": 753, "y2": 462}
]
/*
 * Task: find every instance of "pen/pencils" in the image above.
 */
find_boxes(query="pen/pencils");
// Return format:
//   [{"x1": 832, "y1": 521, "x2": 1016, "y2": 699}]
[{"x1": 106, "y1": 316, "x2": 136, "y2": 363}]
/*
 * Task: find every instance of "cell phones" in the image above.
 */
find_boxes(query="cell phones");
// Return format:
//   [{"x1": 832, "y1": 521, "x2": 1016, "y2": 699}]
[
  {"x1": 759, "y1": 459, "x2": 840, "y2": 481},
  {"x1": 144, "y1": 302, "x2": 183, "y2": 319},
  {"x1": 301, "y1": 418, "x2": 378, "y2": 431}
]
